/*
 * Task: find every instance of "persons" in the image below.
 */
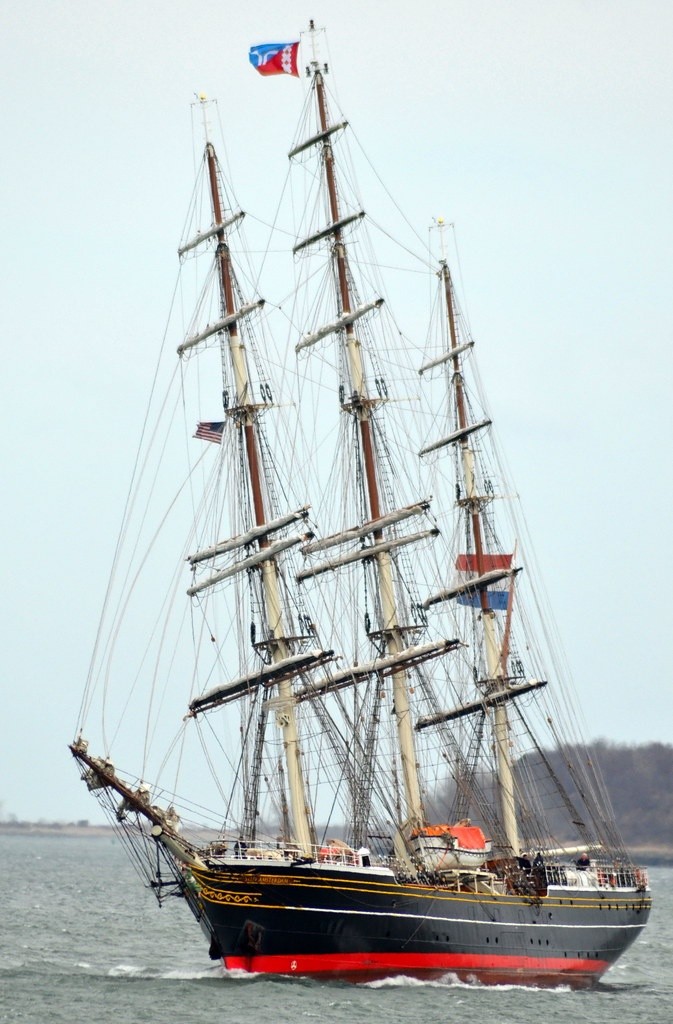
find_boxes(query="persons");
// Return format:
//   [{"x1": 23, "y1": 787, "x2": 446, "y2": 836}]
[
  {"x1": 533, "y1": 853, "x2": 543, "y2": 867},
  {"x1": 514, "y1": 854, "x2": 532, "y2": 874},
  {"x1": 234, "y1": 836, "x2": 247, "y2": 859},
  {"x1": 576, "y1": 853, "x2": 590, "y2": 871}
]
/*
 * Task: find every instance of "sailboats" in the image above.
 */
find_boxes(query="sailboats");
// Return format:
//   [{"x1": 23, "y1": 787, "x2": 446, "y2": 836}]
[{"x1": 69, "y1": 16, "x2": 655, "y2": 989}]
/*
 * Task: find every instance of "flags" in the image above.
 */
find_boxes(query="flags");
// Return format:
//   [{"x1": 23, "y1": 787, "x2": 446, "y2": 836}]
[
  {"x1": 191, "y1": 421, "x2": 226, "y2": 445},
  {"x1": 249, "y1": 41, "x2": 301, "y2": 78},
  {"x1": 455, "y1": 554, "x2": 513, "y2": 610}
]
